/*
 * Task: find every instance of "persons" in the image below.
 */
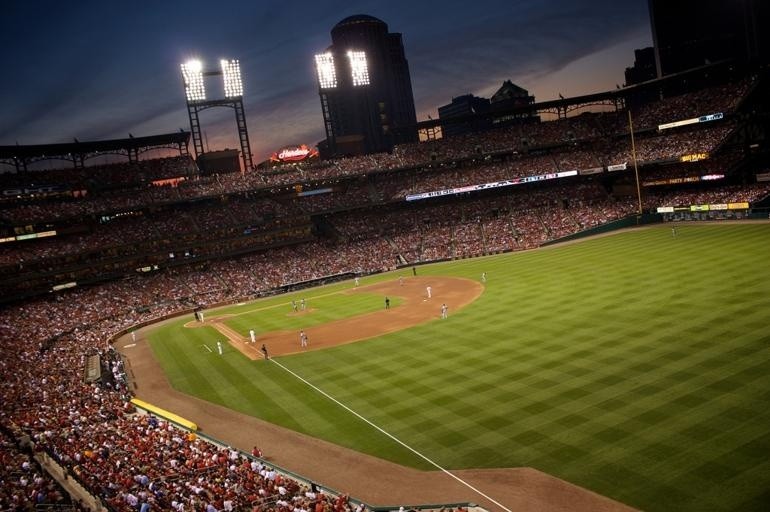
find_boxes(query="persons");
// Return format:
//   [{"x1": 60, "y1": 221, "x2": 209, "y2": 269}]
[
  {"x1": 260, "y1": 343, "x2": 270, "y2": 360},
  {"x1": 1, "y1": 446, "x2": 484, "y2": 512},
  {"x1": 1, "y1": 329, "x2": 210, "y2": 447},
  {"x1": 0, "y1": 66, "x2": 769, "y2": 329},
  {"x1": 217, "y1": 340, "x2": 223, "y2": 355},
  {"x1": 299, "y1": 331, "x2": 308, "y2": 347},
  {"x1": 249, "y1": 329, "x2": 257, "y2": 343}
]
